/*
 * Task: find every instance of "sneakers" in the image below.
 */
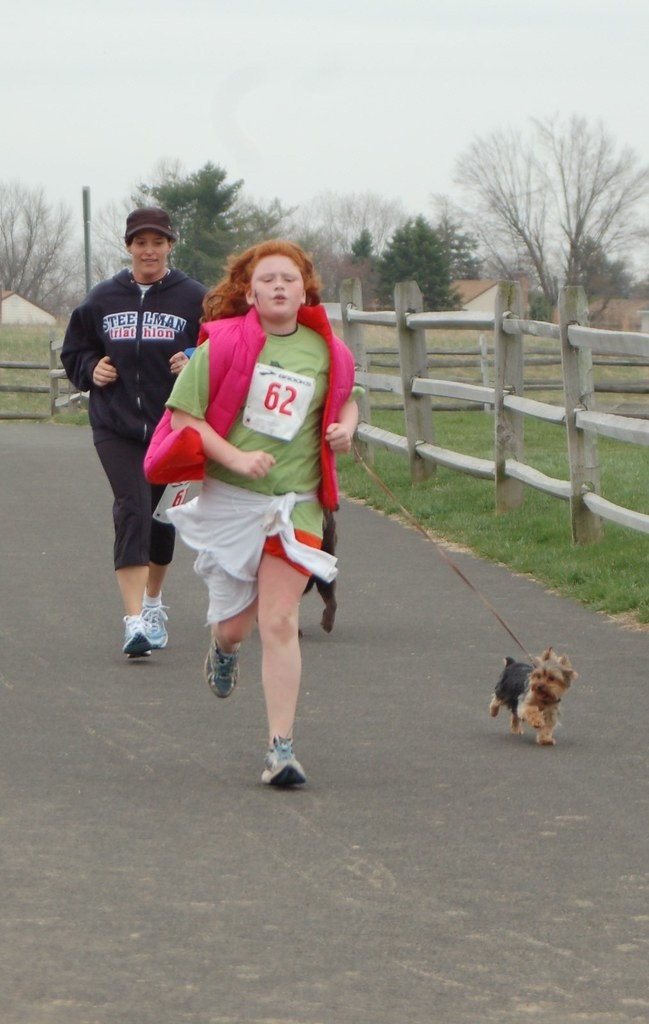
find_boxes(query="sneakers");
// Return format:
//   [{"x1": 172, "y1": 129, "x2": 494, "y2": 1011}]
[
  {"x1": 122, "y1": 615, "x2": 153, "y2": 659},
  {"x1": 204, "y1": 630, "x2": 239, "y2": 699},
  {"x1": 262, "y1": 733, "x2": 307, "y2": 785},
  {"x1": 142, "y1": 603, "x2": 170, "y2": 650}
]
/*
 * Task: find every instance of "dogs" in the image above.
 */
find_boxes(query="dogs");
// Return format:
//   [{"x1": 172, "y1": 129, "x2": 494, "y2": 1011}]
[
  {"x1": 489, "y1": 647, "x2": 577, "y2": 746},
  {"x1": 256, "y1": 503, "x2": 340, "y2": 638}
]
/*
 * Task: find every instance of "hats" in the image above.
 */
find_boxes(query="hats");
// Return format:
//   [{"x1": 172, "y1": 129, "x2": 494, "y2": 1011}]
[{"x1": 125, "y1": 207, "x2": 176, "y2": 245}]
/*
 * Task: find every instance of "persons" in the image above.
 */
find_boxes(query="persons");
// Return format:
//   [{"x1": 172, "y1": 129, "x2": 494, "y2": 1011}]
[
  {"x1": 144, "y1": 238, "x2": 358, "y2": 784},
  {"x1": 60, "y1": 207, "x2": 212, "y2": 658}
]
